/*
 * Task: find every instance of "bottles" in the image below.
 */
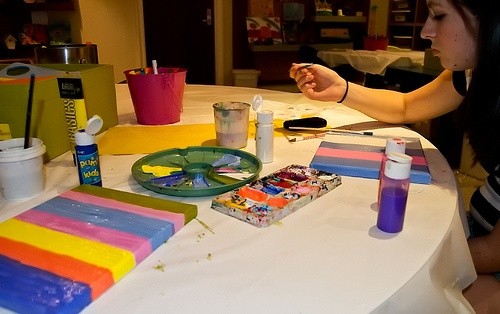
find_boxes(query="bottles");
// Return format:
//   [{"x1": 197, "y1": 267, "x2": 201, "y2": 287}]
[
  {"x1": 376, "y1": 150, "x2": 412, "y2": 232},
  {"x1": 74, "y1": 129, "x2": 102, "y2": 187},
  {"x1": 256, "y1": 111, "x2": 274, "y2": 162}
]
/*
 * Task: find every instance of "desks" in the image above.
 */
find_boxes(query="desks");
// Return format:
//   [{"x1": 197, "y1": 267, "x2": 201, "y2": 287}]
[
  {"x1": 302, "y1": 43, "x2": 425, "y2": 65},
  {"x1": 386, "y1": 66, "x2": 468, "y2": 170},
  {"x1": 0, "y1": 84, "x2": 459, "y2": 314}
]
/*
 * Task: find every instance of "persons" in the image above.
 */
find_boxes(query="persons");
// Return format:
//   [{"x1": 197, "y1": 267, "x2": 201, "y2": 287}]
[{"x1": 289, "y1": 0, "x2": 500, "y2": 314}]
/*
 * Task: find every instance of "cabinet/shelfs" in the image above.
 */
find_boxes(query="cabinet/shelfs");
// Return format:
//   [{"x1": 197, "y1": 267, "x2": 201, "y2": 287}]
[
  {"x1": 0, "y1": 0, "x2": 78, "y2": 64},
  {"x1": 232, "y1": 0, "x2": 432, "y2": 81}
]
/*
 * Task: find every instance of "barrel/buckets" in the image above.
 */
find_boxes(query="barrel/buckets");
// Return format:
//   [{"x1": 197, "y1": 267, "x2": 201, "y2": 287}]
[
  {"x1": 0, "y1": 137, "x2": 47, "y2": 204},
  {"x1": 123, "y1": 67, "x2": 186, "y2": 125}
]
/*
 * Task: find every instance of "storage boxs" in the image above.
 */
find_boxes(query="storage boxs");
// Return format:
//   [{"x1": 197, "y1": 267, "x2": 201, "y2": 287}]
[
  {"x1": 423, "y1": 49, "x2": 445, "y2": 74},
  {"x1": 231, "y1": 69, "x2": 261, "y2": 88},
  {"x1": 0, "y1": 64, "x2": 118, "y2": 163}
]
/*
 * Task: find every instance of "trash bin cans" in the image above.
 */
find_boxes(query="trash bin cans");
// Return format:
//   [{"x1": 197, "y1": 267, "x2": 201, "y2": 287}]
[{"x1": 232, "y1": 69, "x2": 261, "y2": 88}]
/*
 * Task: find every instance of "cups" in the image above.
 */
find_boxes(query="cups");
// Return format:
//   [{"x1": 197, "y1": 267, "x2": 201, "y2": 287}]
[{"x1": 212, "y1": 101, "x2": 250, "y2": 149}]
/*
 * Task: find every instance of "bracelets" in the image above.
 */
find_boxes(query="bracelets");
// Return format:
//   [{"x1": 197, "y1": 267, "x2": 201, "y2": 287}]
[{"x1": 337, "y1": 80, "x2": 348, "y2": 103}]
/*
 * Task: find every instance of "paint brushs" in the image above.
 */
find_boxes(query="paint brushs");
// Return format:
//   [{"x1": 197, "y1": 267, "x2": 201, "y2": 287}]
[
  {"x1": 287, "y1": 65, "x2": 312, "y2": 72},
  {"x1": 128, "y1": 60, "x2": 177, "y2": 74},
  {"x1": 289, "y1": 127, "x2": 373, "y2": 136},
  {"x1": 23, "y1": 73, "x2": 36, "y2": 150},
  {"x1": 366, "y1": 33, "x2": 388, "y2": 51}
]
